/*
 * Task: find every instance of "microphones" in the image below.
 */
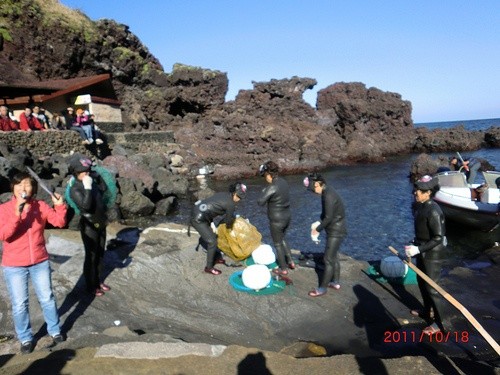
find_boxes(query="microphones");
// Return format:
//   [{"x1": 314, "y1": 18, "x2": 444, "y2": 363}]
[{"x1": 20, "y1": 192, "x2": 27, "y2": 213}]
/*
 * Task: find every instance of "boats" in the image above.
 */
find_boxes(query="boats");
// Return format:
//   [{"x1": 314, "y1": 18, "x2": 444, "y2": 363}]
[{"x1": 429, "y1": 169, "x2": 500, "y2": 228}]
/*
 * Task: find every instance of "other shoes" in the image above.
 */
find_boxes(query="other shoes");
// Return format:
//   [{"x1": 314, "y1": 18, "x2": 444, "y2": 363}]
[
  {"x1": 82, "y1": 139, "x2": 90, "y2": 144},
  {"x1": 330, "y1": 283, "x2": 340, "y2": 289},
  {"x1": 20, "y1": 341, "x2": 32, "y2": 354},
  {"x1": 95, "y1": 139, "x2": 103, "y2": 144},
  {"x1": 274, "y1": 268, "x2": 288, "y2": 275},
  {"x1": 88, "y1": 138, "x2": 94, "y2": 143},
  {"x1": 423, "y1": 321, "x2": 451, "y2": 335},
  {"x1": 95, "y1": 289, "x2": 104, "y2": 296},
  {"x1": 411, "y1": 308, "x2": 434, "y2": 317},
  {"x1": 215, "y1": 258, "x2": 225, "y2": 263},
  {"x1": 308, "y1": 287, "x2": 327, "y2": 297},
  {"x1": 205, "y1": 266, "x2": 222, "y2": 275},
  {"x1": 289, "y1": 262, "x2": 295, "y2": 269},
  {"x1": 50, "y1": 333, "x2": 63, "y2": 343},
  {"x1": 99, "y1": 284, "x2": 110, "y2": 291}
]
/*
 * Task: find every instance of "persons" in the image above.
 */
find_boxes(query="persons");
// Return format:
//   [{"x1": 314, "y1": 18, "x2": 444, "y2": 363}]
[
  {"x1": 403, "y1": 173, "x2": 448, "y2": 336},
  {"x1": 256, "y1": 161, "x2": 295, "y2": 274},
  {"x1": 189, "y1": 182, "x2": 248, "y2": 275},
  {"x1": 68, "y1": 152, "x2": 110, "y2": 296},
  {"x1": 0, "y1": 106, "x2": 104, "y2": 145},
  {"x1": 458, "y1": 157, "x2": 489, "y2": 202},
  {"x1": 451, "y1": 157, "x2": 469, "y2": 180},
  {"x1": 303, "y1": 173, "x2": 346, "y2": 296},
  {"x1": 0, "y1": 172, "x2": 68, "y2": 354}
]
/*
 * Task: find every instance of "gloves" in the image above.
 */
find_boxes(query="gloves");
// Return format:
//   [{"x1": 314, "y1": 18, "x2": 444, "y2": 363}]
[
  {"x1": 311, "y1": 231, "x2": 321, "y2": 245},
  {"x1": 404, "y1": 245, "x2": 420, "y2": 258},
  {"x1": 82, "y1": 175, "x2": 93, "y2": 190},
  {"x1": 211, "y1": 222, "x2": 218, "y2": 235},
  {"x1": 311, "y1": 221, "x2": 321, "y2": 230}
]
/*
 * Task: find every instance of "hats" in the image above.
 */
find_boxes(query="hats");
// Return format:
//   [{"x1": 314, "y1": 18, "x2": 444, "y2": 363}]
[
  {"x1": 448, "y1": 156, "x2": 456, "y2": 163},
  {"x1": 303, "y1": 176, "x2": 314, "y2": 191},
  {"x1": 415, "y1": 174, "x2": 439, "y2": 189},
  {"x1": 71, "y1": 155, "x2": 93, "y2": 171},
  {"x1": 258, "y1": 161, "x2": 279, "y2": 177},
  {"x1": 234, "y1": 181, "x2": 248, "y2": 199},
  {"x1": 77, "y1": 109, "x2": 84, "y2": 114},
  {"x1": 85, "y1": 110, "x2": 90, "y2": 115}
]
